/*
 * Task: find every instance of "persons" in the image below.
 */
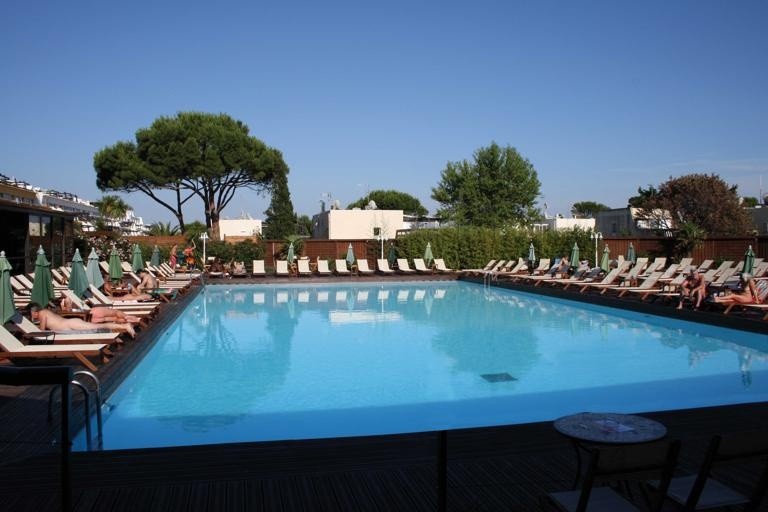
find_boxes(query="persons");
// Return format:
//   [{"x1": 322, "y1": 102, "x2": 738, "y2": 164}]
[
  {"x1": 169, "y1": 242, "x2": 177, "y2": 273},
  {"x1": 714, "y1": 274, "x2": 759, "y2": 304},
  {"x1": 181, "y1": 239, "x2": 197, "y2": 271},
  {"x1": 209, "y1": 257, "x2": 246, "y2": 273},
  {"x1": 558, "y1": 255, "x2": 569, "y2": 271},
  {"x1": 675, "y1": 270, "x2": 705, "y2": 310}
]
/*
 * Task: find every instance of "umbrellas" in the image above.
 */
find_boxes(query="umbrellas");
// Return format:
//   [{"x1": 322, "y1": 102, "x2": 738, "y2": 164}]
[
  {"x1": 286, "y1": 292, "x2": 297, "y2": 322},
  {"x1": 528, "y1": 238, "x2": 536, "y2": 263},
  {"x1": 423, "y1": 288, "x2": 435, "y2": 321},
  {"x1": 424, "y1": 242, "x2": 434, "y2": 269},
  {"x1": 599, "y1": 243, "x2": 611, "y2": 273},
  {"x1": 0, "y1": 244, "x2": 161, "y2": 337},
  {"x1": 570, "y1": 242, "x2": 580, "y2": 268},
  {"x1": 345, "y1": 289, "x2": 356, "y2": 320},
  {"x1": 388, "y1": 242, "x2": 397, "y2": 268},
  {"x1": 741, "y1": 244, "x2": 755, "y2": 275},
  {"x1": 627, "y1": 242, "x2": 635, "y2": 265},
  {"x1": 286, "y1": 242, "x2": 295, "y2": 264},
  {"x1": 346, "y1": 242, "x2": 354, "y2": 266}
]
